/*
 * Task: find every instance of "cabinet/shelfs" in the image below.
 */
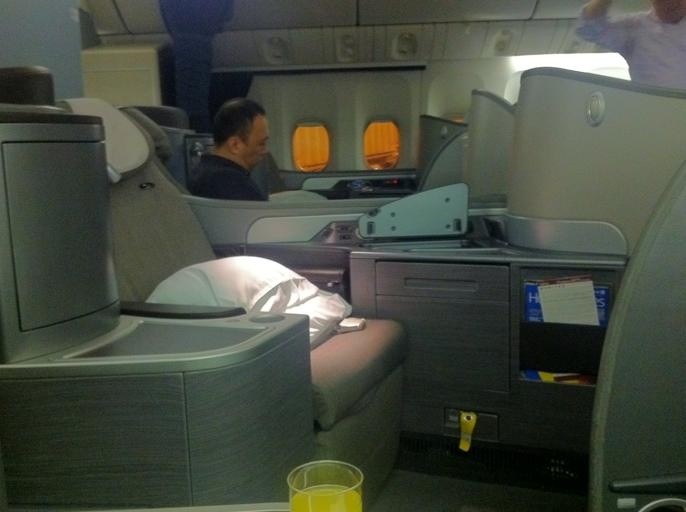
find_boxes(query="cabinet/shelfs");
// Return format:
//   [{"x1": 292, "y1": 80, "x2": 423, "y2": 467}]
[{"x1": 348, "y1": 239, "x2": 631, "y2": 470}]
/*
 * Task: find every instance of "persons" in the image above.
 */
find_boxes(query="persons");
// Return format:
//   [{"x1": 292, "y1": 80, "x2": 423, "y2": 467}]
[{"x1": 186, "y1": 96, "x2": 274, "y2": 201}]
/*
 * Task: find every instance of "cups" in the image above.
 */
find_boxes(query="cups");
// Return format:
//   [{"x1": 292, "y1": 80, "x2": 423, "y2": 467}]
[{"x1": 287, "y1": 460, "x2": 364, "y2": 512}]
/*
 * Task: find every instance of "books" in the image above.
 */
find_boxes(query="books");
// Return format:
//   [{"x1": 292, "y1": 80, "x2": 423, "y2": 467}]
[
  {"x1": 520, "y1": 280, "x2": 619, "y2": 389},
  {"x1": 536, "y1": 276, "x2": 601, "y2": 326}
]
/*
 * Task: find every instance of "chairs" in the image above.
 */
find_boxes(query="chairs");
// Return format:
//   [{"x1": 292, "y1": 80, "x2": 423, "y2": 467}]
[{"x1": 55, "y1": 96, "x2": 407, "y2": 510}]
